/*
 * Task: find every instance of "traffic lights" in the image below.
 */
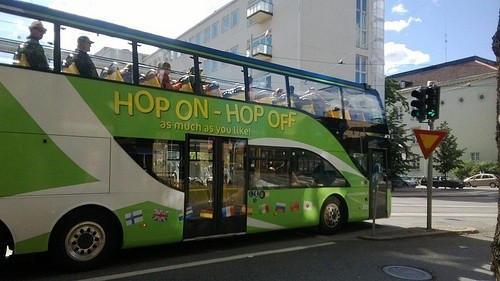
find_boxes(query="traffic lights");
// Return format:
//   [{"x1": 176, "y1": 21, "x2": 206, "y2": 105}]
[
  {"x1": 411, "y1": 87, "x2": 425, "y2": 121},
  {"x1": 425, "y1": 86, "x2": 439, "y2": 120}
]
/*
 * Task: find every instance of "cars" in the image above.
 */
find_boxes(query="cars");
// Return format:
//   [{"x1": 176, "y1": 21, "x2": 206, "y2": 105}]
[
  {"x1": 463, "y1": 173, "x2": 499, "y2": 188},
  {"x1": 421, "y1": 175, "x2": 465, "y2": 189},
  {"x1": 396, "y1": 174, "x2": 417, "y2": 188}
]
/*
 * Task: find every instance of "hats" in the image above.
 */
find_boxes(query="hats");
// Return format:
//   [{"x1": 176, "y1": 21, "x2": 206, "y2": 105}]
[
  {"x1": 190, "y1": 67, "x2": 203, "y2": 72},
  {"x1": 29, "y1": 21, "x2": 47, "y2": 31},
  {"x1": 78, "y1": 36, "x2": 94, "y2": 43}
]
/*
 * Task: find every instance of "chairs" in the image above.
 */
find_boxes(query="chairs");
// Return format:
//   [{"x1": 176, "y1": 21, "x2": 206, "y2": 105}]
[{"x1": 13, "y1": 45, "x2": 351, "y2": 120}]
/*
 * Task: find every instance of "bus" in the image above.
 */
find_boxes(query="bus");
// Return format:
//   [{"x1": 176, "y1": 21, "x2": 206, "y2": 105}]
[{"x1": 0, "y1": 0, "x2": 392, "y2": 275}]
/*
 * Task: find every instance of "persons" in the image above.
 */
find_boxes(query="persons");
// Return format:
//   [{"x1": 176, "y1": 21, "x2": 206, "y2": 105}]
[
  {"x1": 13, "y1": 21, "x2": 52, "y2": 71},
  {"x1": 109, "y1": 61, "x2": 132, "y2": 82},
  {"x1": 72, "y1": 35, "x2": 102, "y2": 79},
  {"x1": 156, "y1": 61, "x2": 184, "y2": 92},
  {"x1": 289, "y1": 85, "x2": 303, "y2": 109},
  {"x1": 275, "y1": 88, "x2": 283, "y2": 96}
]
